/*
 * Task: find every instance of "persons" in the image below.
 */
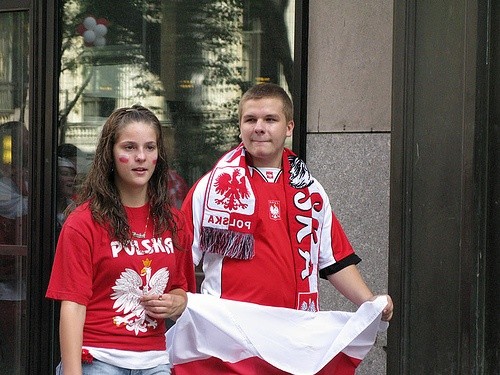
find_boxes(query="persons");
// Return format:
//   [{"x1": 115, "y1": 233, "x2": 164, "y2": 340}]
[
  {"x1": 57, "y1": 144, "x2": 85, "y2": 206},
  {"x1": 0, "y1": 121, "x2": 31, "y2": 375},
  {"x1": 44, "y1": 105, "x2": 197, "y2": 375},
  {"x1": 180, "y1": 82, "x2": 394, "y2": 322},
  {"x1": 57, "y1": 157, "x2": 77, "y2": 227}
]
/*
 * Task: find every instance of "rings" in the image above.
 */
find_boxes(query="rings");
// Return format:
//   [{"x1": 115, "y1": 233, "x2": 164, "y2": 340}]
[{"x1": 158, "y1": 292, "x2": 163, "y2": 300}]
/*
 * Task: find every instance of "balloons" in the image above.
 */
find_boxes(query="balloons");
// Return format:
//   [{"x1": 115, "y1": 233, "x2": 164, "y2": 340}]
[{"x1": 77, "y1": 17, "x2": 109, "y2": 50}]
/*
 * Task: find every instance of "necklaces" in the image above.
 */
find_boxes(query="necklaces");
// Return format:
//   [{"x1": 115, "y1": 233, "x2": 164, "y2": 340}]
[{"x1": 129, "y1": 202, "x2": 151, "y2": 237}]
